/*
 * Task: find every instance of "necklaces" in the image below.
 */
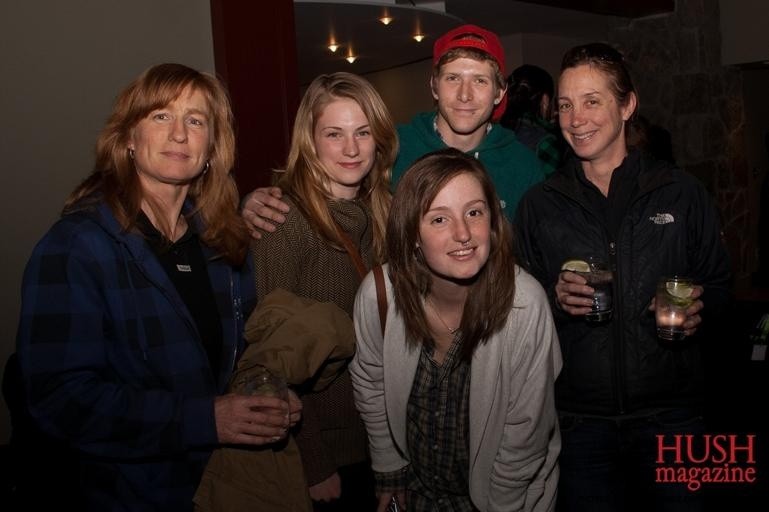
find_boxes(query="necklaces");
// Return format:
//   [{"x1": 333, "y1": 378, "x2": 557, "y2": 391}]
[{"x1": 426, "y1": 292, "x2": 461, "y2": 335}]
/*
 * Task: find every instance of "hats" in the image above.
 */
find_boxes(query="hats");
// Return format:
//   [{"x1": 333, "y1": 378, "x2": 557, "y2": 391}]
[{"x1": 434, "y1": 25, "x2": 508, "y2": 119}]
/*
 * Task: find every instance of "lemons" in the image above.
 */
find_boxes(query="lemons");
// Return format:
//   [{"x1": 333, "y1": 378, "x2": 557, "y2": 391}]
[
  {"x1": 562, "y1": 260, "x2": 590, "y2": 272},
  {"x1": 666, "y1": 282, "x2": 694, "y2": 298}
]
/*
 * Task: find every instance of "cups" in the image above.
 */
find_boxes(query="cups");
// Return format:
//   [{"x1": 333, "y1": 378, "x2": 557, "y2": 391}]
[
  {"x1": 245, "y1": 378, "x2": 290, "y2": 439},
  {"x1": 573, "y1": 256, "x2": 614, "y2": 322},
  {"x1": 655, "y1": 273, "x2": 705, "y2": 342}
]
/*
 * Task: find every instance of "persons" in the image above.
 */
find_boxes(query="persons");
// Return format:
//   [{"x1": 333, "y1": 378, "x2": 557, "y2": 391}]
[
  {"x1": 1, "y1": 60, "x2": 304, "y2": 510},
  {"x1": 500, "y1": 63, "x2": 562, "y2": 165},
  {"x1": 507, "y1": 38, "x2": 732, "y2": 510},
  {"x1": 240, "y1": 24, "x2": 550, "y2": 248},
  {"x1": 250, "y1": 69, "x2": 401, "y2": 510},
  {"x1": 346, "y1": 146, "x2": 562, "y2": 512}
]
